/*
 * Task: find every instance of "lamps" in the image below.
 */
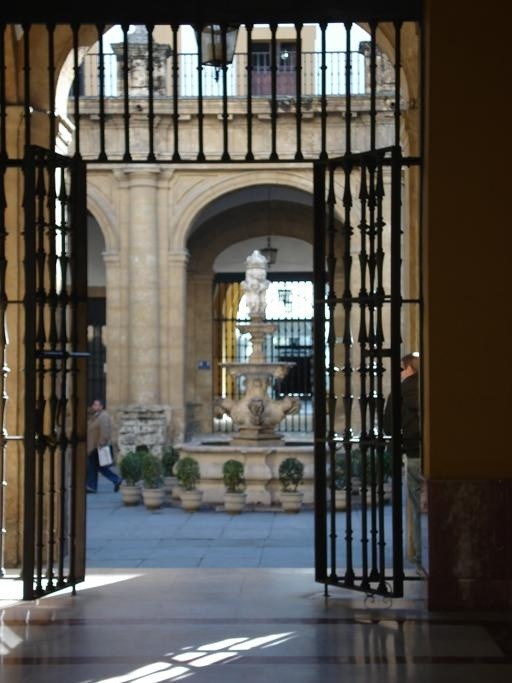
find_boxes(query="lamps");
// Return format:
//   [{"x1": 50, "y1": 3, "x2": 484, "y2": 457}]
[
  {"x1": 192, "y1": 23, "x2": 240, "y2": 81},
  {"x1": 260, "y1": 235, "x2": 279, "y2": 269}
]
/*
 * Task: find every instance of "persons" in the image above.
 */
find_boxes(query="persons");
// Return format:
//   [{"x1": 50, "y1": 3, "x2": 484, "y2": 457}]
[
  {"x1": 382, "y1": 353, "x2": 420, "y2": 563},
  {"x1": 84, "y1": 400, "x2": 123, "y2": 492}
]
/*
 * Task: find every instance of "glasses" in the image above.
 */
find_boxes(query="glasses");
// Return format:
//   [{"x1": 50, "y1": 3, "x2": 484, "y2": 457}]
[{"x1": 400, "y1": 367, "x2": 404, "y2": 372}]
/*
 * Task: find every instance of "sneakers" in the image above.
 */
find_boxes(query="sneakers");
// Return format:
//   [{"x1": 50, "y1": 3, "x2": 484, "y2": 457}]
[{"x1": 85, "y1": 478, "x2": 123, "y2": 493}]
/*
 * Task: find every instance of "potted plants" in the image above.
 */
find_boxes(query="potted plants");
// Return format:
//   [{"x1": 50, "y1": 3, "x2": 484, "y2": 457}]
[{"x1": 121, "y1": 450, "x2": 394, "y2": 512}]
